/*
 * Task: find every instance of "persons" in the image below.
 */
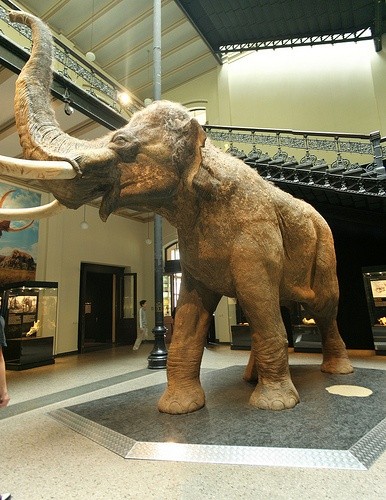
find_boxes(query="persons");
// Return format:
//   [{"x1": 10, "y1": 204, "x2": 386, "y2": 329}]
[
  {"x1": 133, "y1": 300, "x2": 148, "y2": 351},
  {"x1": 0, "y1": 313, "x2": 11, "y2": 408}
]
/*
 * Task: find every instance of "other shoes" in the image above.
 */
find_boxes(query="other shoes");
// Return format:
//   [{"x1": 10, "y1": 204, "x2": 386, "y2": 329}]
[{"x1": 0, "y1": 492, "x2": 11, "y2": 500}]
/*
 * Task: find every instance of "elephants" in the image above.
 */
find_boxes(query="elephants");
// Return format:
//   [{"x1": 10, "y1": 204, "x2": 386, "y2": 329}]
[{"x1": 0, "y1": 9, "x2": 355, "y2": 415}]
